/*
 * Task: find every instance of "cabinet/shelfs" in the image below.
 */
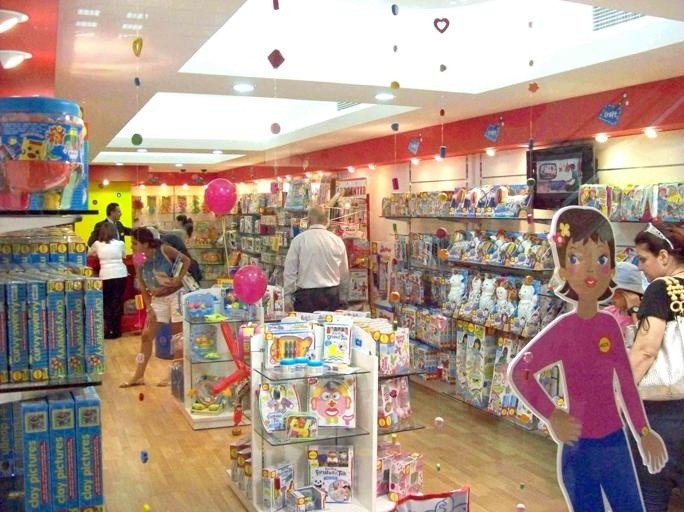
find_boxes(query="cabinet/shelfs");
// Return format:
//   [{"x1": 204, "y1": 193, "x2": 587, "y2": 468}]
[
  {"x1": 0, "y1": 209, "x2": 105, "y2": 408},
  {"x1": 241, "y1": 193, "x2": 372, "y2": 314},
  {"x1": 228, "y1": 312, "x2": 429, "y2": 511},
  {"x1": 170, "y1": 283, "x2": 285, "y2": 430},
  {"x1": 379, "y1": 213, "x2": 555, "y2": 433}
]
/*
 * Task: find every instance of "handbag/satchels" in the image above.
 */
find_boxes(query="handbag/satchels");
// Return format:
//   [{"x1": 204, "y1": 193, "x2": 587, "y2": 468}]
[{"x1": 626, "y1": 275, "x2": 684, "y2": 401}]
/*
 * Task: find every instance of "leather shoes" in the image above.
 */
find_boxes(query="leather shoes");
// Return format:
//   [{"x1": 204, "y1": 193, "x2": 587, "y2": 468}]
[{"x1": 104, "y1": 330, "x2": 121, "y2": 341}]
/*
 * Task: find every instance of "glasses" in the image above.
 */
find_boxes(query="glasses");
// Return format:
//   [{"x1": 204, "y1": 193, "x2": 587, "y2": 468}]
[{"x1": 643, "y1": 220, "x2": 675, "y2": 250}]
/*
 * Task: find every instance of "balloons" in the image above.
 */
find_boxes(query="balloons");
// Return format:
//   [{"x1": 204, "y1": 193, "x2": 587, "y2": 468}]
[
  {"x1": 234, "y1": 264, "x2": 267, "y2": 324},
  {"x1": 204, "y1": 179, "x2": 238, "y2": 279}
]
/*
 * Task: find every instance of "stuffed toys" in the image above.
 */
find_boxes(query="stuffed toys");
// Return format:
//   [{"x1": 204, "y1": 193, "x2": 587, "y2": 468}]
[
  {"x1": 441, "y1": 271, "x2": 468, "y2": 319},
  {"x1": 466, "y1": 273, "x2": 482, "y2": 310},
  {"x1": 443, "y1": 229, "x2": 555, "y2": 271},
  {"x1": 472, "y1": 277, "x2": 495, "y2": 321},
  {"x1": 518, "y1": 277, "x2": 540, "y2": 327},
  {"x1": 491, "y1": 275, "x2": 518, "y2": 329}
]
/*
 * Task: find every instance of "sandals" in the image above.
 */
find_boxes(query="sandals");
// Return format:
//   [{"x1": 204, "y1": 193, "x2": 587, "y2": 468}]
[
  {"x1": 157, "y1": 379, "x2": 171, "y2": 387},
  {"x1": 118, "y1": 377, "x2": 147, "y2": 388}
]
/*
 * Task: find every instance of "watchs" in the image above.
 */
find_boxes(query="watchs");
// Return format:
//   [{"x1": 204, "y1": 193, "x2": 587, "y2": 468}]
[
  {"x1": 626, "y1": 306, "x2": 639, "y2": 314},
  {"x1": 638, "y1": 426, "x2": 650, "y2": 437},
  {"x1": 145, "y1": 306, "x2": 153, "y2": 312}
]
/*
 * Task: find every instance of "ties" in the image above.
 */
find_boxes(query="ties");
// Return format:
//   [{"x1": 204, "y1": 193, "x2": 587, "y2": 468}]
[{"x1": 113, "y1": 222, "x2": 119, "y2": 240}]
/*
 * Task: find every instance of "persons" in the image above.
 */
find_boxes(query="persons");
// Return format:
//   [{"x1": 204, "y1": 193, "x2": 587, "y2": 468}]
[
  {"x1": 89, "y1": 223, "x2": 129, "y2": 339},
  {"x1": 604, "y1": 262, "x2": 650, "y2": 353},
  {"x1": 139, "y1": 226, "x2": 202, "y2": 283},
  {"x1": 628, "y1": 220, "x2": 683, "y2": 511},
  {"x1": 560, "y1": 164, "x2": 579, "y2": 191},
  {"x1": 87, "y1": 202, "x2": 134, "y2": 247},
  {"x1": 283, "y1": 205, "x2": 350, "y2": 311},
  {"x1": 119, "y1": 227, "x2": 191, "y2": 388},
  {"x1": 176, "y1": 214, "x2": 193, "y2": 242},
  {"x1": 506, "y1": 206, "x2": 668, "y2": 511}
]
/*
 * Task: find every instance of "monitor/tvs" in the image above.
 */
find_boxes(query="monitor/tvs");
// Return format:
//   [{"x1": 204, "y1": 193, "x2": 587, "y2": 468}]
[{"x1": 527, "y1": 143, "x2": 598, "y2": 211}]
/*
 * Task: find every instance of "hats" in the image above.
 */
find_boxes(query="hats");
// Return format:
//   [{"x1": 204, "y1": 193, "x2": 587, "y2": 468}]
[{"x1": 613, "y1": 261, "x2": 645, "y2": 295}]
